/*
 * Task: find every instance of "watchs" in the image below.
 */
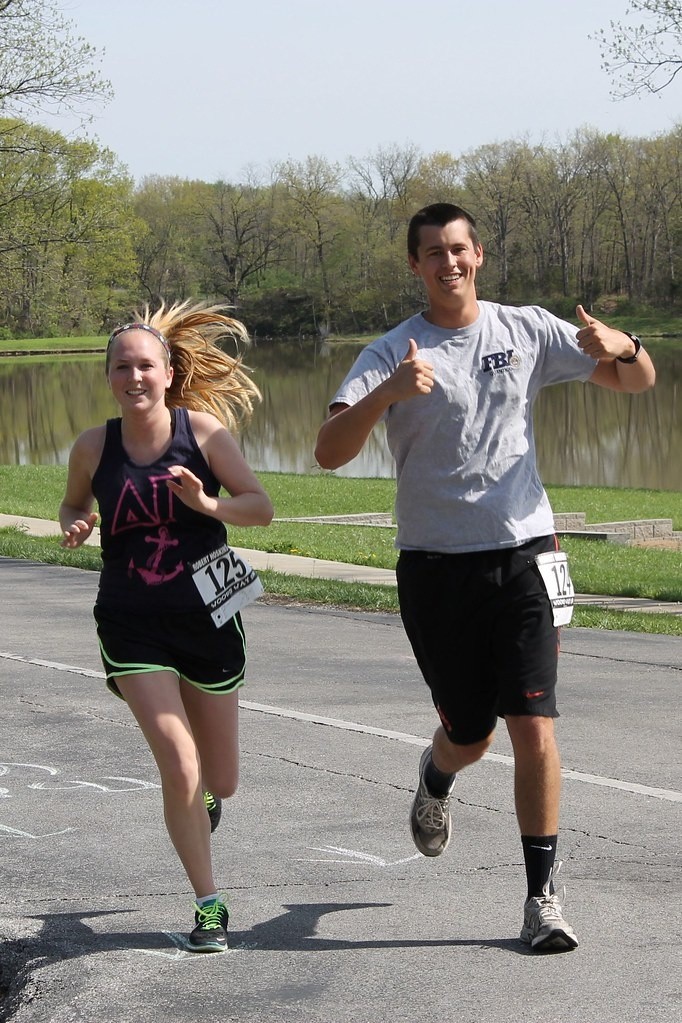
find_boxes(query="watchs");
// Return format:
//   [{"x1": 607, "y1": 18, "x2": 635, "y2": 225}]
[{"x1": 616, "y1": 331, "x2": 642, "y2": 363}]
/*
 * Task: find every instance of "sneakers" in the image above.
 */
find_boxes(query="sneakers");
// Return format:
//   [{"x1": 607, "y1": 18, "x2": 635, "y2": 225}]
[
  {"x1": 202, "y1": 791, "x2": 222, "y2": 833},
  {"x1": 409, "y1": 743, "x2": 458, "y2": 857},
  {"x1": 186, "y1": 893, "x2": 230, "y2": 953},
  {"x1": 518, "y1": 861, "x2": 579, "y2": 953}
]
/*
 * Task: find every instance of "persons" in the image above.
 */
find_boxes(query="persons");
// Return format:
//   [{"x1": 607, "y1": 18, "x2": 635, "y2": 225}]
[
  {"x1": 315, "y1": 203, "x2": 655, "y2": 951},
  {"x1": 60, "y1": 309, "x2": 274, "y2": 952}
]
